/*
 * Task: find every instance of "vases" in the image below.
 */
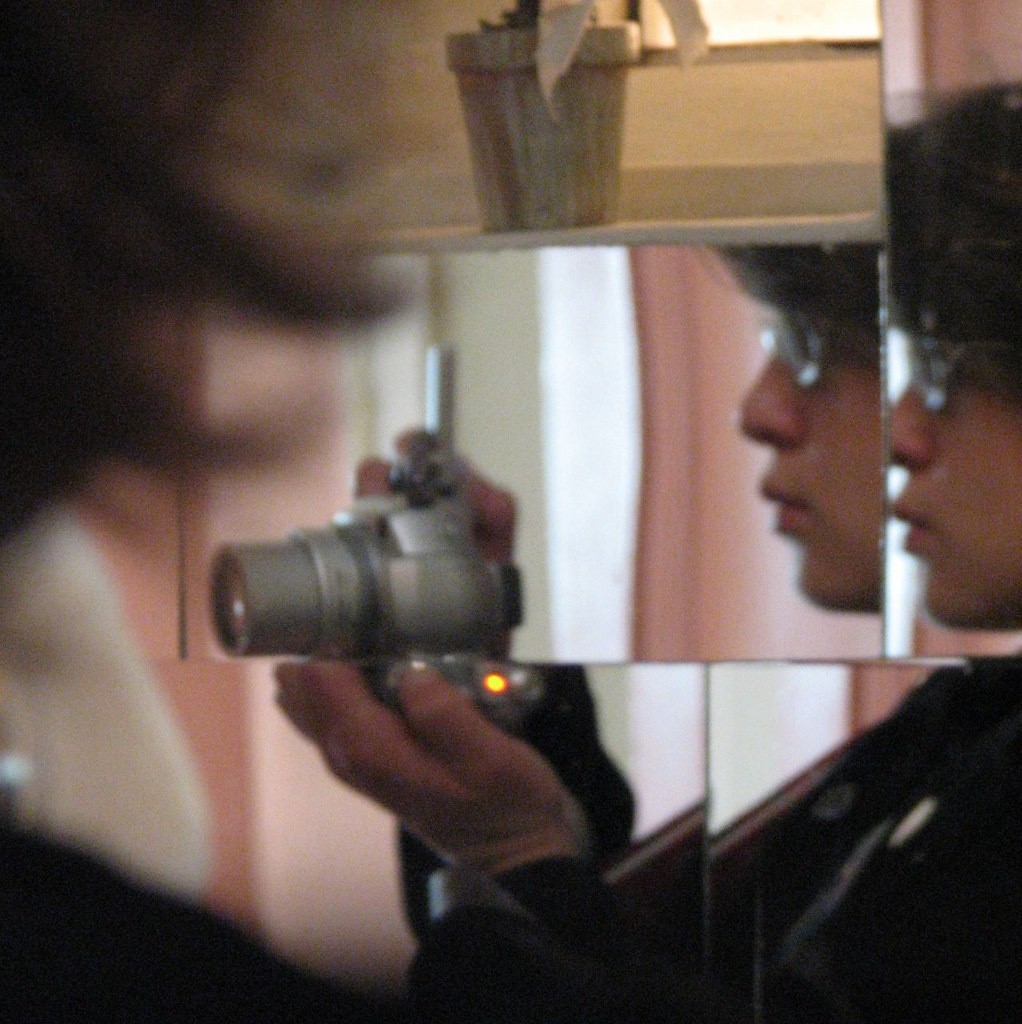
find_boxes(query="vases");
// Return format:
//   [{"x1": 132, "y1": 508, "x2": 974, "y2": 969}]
[{"x1": 446, "y1": 22, "x2": 638, "y2": 230}]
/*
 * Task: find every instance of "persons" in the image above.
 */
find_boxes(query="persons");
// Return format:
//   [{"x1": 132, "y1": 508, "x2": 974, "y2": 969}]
[
  {"x1": 270, "y1": 81, "x2": 1022, "y2": 1024},
  {"x1": 0, "y1": 0, "x2": 341, "y2": 1024},
  {"x1": 364, "y1": 242, "x2": 884, "y2": 647}
]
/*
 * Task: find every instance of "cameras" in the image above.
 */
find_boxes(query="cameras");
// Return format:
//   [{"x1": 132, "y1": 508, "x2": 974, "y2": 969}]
[
  {"x1": 213, "y1": 453, "x2": 521, "y2": 663},
  {"x1": 352, "y1": 655, "x2": 545, "y2": 730}
]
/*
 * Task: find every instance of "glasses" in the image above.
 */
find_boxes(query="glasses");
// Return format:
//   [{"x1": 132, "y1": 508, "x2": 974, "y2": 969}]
[
  {"x1": 763, "y1": 313, "x2": 878, "y2": 388},
  {"x1": 900, "y1": 332, "x2": 1020, "y2": 409}
]
